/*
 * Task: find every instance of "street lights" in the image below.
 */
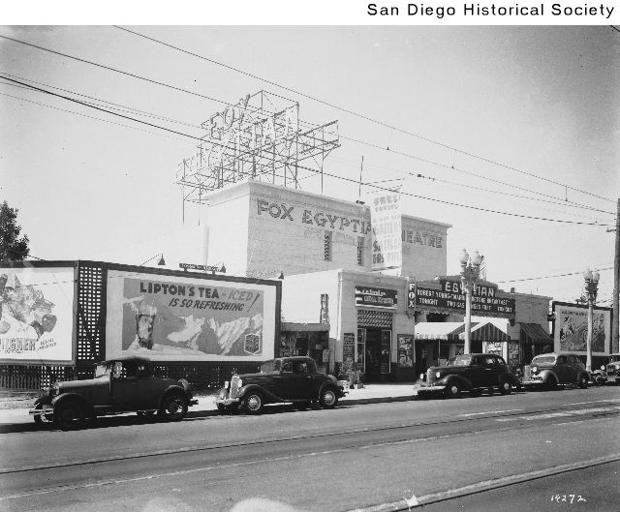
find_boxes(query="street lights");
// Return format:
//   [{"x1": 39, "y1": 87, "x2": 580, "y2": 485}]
[
  {"x1": 459, "y1": 248, "x2": 486, "y2": 354},
  {"x1": 582, "y1": 271, "x2": 600, "y2": 378}
]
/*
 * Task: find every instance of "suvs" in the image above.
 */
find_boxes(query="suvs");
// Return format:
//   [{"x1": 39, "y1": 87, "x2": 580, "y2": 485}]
[
  {"x1": 216, "y1": 356, "x2": 350, "y2": 414},
  {"x1": 28, "y1": 355, "x2": 199, "y2": 430}
]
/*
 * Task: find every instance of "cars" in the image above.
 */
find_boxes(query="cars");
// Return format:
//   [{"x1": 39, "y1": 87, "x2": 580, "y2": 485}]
[
  {"x1": 591, "y1": 353, "x2": 619, "y2": 386},
  {"x1": 414, "y1": 353, "x2": 521, "y2": 397},
  {"x1": 516, "y1": 351, "x2": 589, "y2": 389}
]
